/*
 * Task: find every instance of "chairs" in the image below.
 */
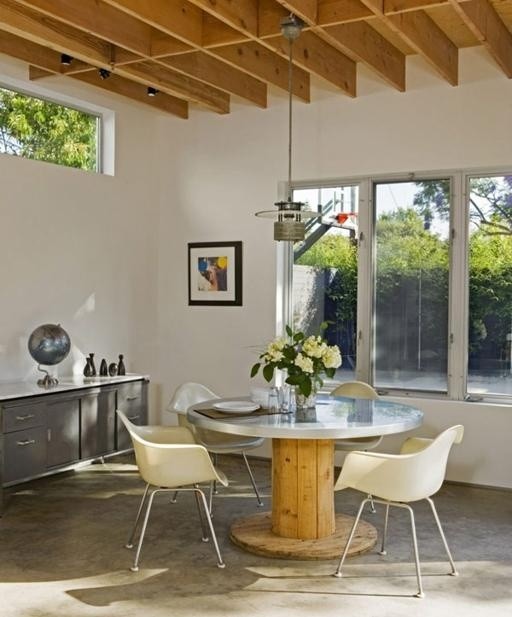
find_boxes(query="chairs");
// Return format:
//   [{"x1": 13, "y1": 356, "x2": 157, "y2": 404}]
[
  {"x1": 330, "y1": 379, "x2": 384, "y2": 514},
  {"x1": 332, "y1": 421, "x2": 465, "y2": 599},
  {"x1": 114, "y1": 405, "x2": 230, "y2": 571},
  {"x1": 165, "y1": 379, "x2": 264, "y2": 518}
]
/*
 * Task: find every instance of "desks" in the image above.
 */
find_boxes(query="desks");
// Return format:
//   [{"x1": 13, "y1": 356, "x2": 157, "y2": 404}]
[{"x1": 186, "y1": 393, "x2": 425, "y2": 562}]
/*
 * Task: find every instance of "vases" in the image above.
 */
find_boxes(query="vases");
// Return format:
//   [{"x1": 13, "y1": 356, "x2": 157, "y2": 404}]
[{"x1": 294, "y1": 375, "x2": 317, "y2": 410}]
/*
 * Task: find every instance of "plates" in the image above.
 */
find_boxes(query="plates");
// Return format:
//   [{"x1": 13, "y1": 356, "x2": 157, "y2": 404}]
[{"x1": 213, "y1": 399, "x2": 261, "y2": 413}]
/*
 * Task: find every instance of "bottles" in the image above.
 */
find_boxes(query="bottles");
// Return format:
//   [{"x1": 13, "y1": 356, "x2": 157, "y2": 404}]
[
  {"x1": 268, "y1": 384, "x2": 297, "y2": 413},
  {"x1": 83, "y1": 351, "x2": 126, "y2": 376},
  {"x1": 268, "y1": 414, "x2": 295, "y2": 429}
]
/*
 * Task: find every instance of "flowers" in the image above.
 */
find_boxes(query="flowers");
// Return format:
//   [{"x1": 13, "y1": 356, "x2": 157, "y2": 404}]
[{"x1": 244, "y1": 319, "x2": 343, "y2": 410}]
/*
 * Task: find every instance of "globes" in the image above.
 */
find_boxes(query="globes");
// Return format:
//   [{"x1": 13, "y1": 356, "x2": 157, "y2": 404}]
[{"x1": 28, "y1": 324, "x2": 71, "y2": 389}]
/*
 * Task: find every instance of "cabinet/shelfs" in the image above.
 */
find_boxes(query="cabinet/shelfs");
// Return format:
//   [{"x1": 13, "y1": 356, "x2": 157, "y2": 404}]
[{"x1": 0, "y1": 373, "x2": 150, "y2": 519}]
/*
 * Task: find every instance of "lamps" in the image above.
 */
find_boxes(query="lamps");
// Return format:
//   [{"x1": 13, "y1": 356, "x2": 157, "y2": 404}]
[
  {"x1": 96, "y1": 68, "x2": 113, "y2": 81},
  {"x1": 147, "y1": 86, "x2": 160, "y2": 96},
  {"x1": 59, "y1": 53, "x2": 74, "y2": 65},
  {"x1": 255, "y1": 11, "x2": 325, "y2": 244}
]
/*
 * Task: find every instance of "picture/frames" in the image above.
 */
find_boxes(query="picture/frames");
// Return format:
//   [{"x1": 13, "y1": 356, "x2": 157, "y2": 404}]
[{"x1": 187, "y1": 239, "x2": 244, "y2": 307}]
